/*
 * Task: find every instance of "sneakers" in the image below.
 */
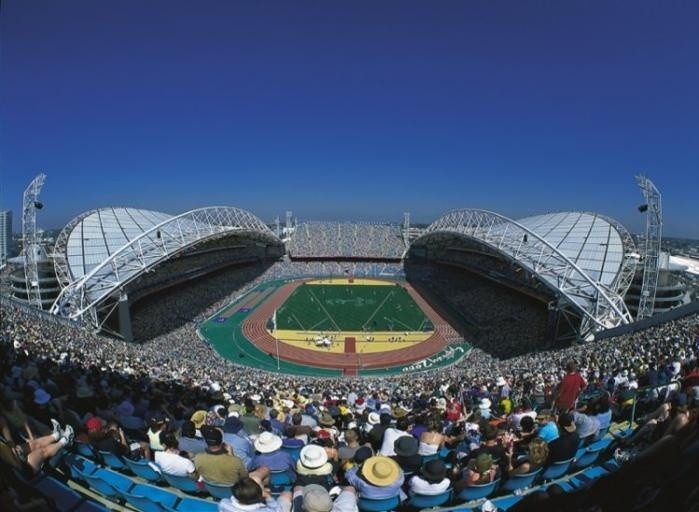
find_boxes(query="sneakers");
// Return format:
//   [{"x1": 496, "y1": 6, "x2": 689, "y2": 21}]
[{"x1": 49, "y1": 418, "x2": 75, "y2": 449}]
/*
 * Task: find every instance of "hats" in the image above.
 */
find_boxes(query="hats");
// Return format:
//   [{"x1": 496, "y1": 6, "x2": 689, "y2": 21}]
[
  {"x1": 298, "y1": 444, "x2": 327, "y2": 469},
  {"x1": 362, "y1": 456, "x2": 400, "y2": 487},
  {"x1": 390, "y1": 409, "x2": 406, "y2": 420},
  {"x1": 206, "y1": 428, "x2": 224, "y2": 447},
  {"x1": 420, "y1": 461, "x2": 445, "y2": 482},
  {"x1": 421, "y1": 376, "x2": 699, "y2": 476},
  {"x1": 394, "y1": 436, "x2": 417, "y2": 456},
  {"x1": 318, "y1": 415, "x2": 336, "y2": 426},
  {"x1": 149, "y1": 410, "x2": 185, "y2": 446},
  {"x1": 0, "y1": 366, "x2": 149, "y2": 431},
  {"x1": 190, "y1": 409, "x2": 207, "y2": 428},
  {"x1": 367, "y1": 412, "x2": 380, "y2": 425},
  {"x1": 224, "y1": 417, "x2": 243, "y2": 434},
  {"x1": 254, "y1": 431, "x2": 283, "y2": 453},
  {"x1": 301, "y1": 483, "x2": 333, "y2": 512},
  {"x1": 150, "y1": 365, "x2": 417, "y2": 408}
]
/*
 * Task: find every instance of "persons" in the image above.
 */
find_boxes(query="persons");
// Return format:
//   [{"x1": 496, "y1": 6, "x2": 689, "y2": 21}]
[{"x1": 0, "y1": 219, "x2": 699, "y2": 509}]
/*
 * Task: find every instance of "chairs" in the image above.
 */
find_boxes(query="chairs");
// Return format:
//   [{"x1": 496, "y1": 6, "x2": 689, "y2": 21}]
[{"x1": 0, "y1": 371, "x2": 699, "y2": 512}]
